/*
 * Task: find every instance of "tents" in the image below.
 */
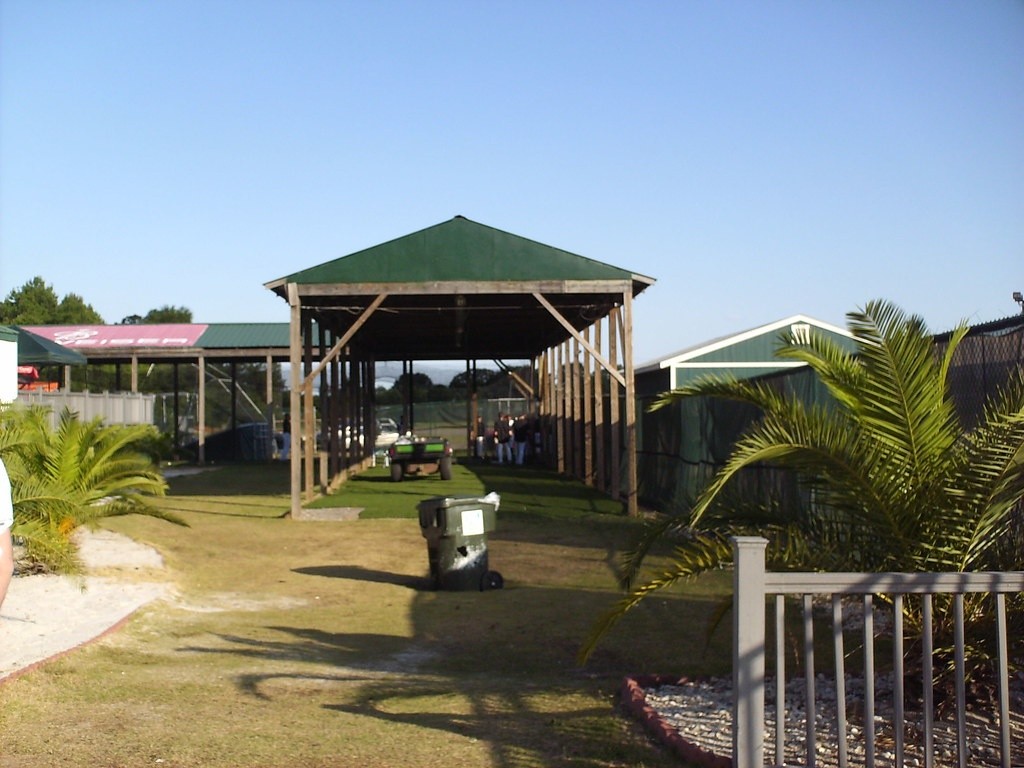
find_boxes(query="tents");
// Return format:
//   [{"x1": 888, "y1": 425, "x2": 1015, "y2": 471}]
[
  {"x1": 18, "y1": 363, "x2": 39, "y2": 391},
  {"x1": 6, "y1": 323, "x2": 89, "y2": 392}
]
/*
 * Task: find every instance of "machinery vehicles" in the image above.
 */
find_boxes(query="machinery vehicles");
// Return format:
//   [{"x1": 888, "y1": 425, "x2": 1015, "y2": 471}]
[{"x1": 389, "y1": 435, "x2": 454, "y2": 481}]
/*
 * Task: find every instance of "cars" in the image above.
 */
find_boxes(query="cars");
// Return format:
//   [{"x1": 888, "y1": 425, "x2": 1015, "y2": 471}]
[{"x1": 238, "y1": 412, "x2": 411, "y2": 451}]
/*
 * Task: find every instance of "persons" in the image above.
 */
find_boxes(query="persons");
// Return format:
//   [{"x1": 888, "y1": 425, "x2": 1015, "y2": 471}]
[
  {"x1": 398, "y1": 415, "x2": 413, "y2": 439},
  {"x1": 494, "y1": 411, "x2": 545, "y2": 465},
  {"x1": 476, "y1": 416, "x2": 485, "y2": 459},
  {"x1": 0, "y1": 457, "x2": 16, "y2": 612},
  {"x1": 280, "y1": 413, "x2": 291, "y2": 460}
]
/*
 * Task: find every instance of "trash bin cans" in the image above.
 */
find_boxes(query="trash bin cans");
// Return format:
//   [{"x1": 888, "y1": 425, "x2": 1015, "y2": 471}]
[{"x1": 416, "y1": 495, "x2": 504, "y2": 592}]
